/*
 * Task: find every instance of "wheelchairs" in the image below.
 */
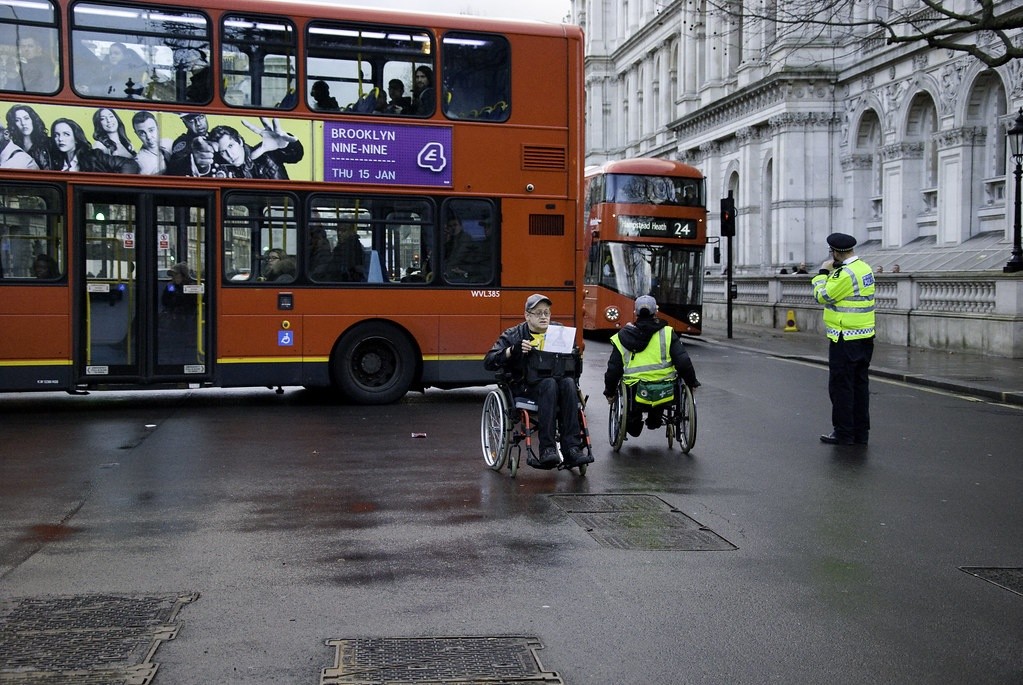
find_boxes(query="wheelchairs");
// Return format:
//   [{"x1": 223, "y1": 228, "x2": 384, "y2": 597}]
[
  {"x1": 608, "y1": 372, "x2": 698, "y2": 454},
  {"x1": 480, "y1": 364, "x2": 596, "y2": 478}
]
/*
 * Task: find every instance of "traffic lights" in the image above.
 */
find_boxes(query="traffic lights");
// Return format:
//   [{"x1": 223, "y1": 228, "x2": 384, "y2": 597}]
[
  {"x1": 721, "y1": 198, "x2": 735, "y2": 236},
  {"x1": 415, "y1": 254, "x2": 419, "y2": 261},
  {"x1": 92, "y1": 203, "x2": 110, "y2": 224}
]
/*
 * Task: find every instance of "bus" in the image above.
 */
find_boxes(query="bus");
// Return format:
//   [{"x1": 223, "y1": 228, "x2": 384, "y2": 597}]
[
  {"x1": 0, "y1": 0, "x2": 587, "y2": 409},
  {"x1": 579, "y1": 159, "x2": 720, "y2": 343}
]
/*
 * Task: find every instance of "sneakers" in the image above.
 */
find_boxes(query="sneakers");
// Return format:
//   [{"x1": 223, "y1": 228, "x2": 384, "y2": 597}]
[
  {"x1": 539, "y1": 446, "x2": 562, "y2": 465},
  {"x1": 562, "y1": 448, "x2": 591, "y2": 466}
]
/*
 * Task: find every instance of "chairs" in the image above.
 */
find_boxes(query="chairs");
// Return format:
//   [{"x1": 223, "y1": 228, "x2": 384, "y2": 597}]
[{"x1": 277, "y1": 80, "x2": 509, "y2": 116}]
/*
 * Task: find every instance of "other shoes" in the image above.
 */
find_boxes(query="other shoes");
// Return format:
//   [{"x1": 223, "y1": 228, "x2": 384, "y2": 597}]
[
  {"x1": 616, "y1": 414, "x2": 644, "y2": 438},
  {"x1": 646, "y1": 412, "x2": 663, "y2": 430}
]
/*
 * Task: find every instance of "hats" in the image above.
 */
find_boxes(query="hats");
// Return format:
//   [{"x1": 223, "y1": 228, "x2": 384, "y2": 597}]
[
  {"x1": 173, "y1": 110, "x2": 199, "y2": 121},
  {"x1": 827, "y1": 233, "x2": 857, "y2": 252},
  {"x1": 526, "y1": 293, "x2": 553, "y2": 311},
  {"x1": 167, "y1": 261, "x2": 188, "y2": 278},
  {"x1": 634, "y1": 295, "x2": 659, "y2": 315}
]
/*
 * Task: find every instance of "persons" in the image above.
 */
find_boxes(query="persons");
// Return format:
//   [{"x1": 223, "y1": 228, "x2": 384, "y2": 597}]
[
  {"x1": 32, "y1": 216, "x2": 494, "y2": 362},
  {"x1": 706, "y1": 262, "x2": 900, "y2": 274},
  {"x1": 485, "y1": 293, "x2": 587, "y2": 467},
  {"x1": 0, "y1": 105, "x2": 305, "y2": 180},
  {"x1": 0, "y1": 33, "x2": 437, "y2": 116},
  {"x1": 604, "y1": 295, "x2": 702, "y2": 439},
  {"x1": 812, "y1": 233, "x2": 876, "y2": 448}
]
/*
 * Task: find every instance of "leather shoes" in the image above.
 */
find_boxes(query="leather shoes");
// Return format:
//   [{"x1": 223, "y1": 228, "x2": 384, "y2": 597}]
[
  {"x1": 855, "y1": 432, "x2": 868, "y2": 445},
  {"x1": 819, "y1": 431, "x2": 854, "y2": 445}
]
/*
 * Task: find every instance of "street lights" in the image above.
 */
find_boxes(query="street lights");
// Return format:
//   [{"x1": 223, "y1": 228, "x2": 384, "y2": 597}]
[{"x1": 1003, "y1": 106, "x2": 1023, "y2": 274}]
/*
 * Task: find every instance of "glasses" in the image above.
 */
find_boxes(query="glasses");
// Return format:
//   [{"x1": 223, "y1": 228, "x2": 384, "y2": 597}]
[
  {"x1": 265, "y1": 256, "x2": 280, "y2": 260},
  {"x1": 526, "y1": 310, "x2": 551, "y2": 317},
  {"x1": 388, "y1": 85, "x2": 400, "y2": 90}
]
